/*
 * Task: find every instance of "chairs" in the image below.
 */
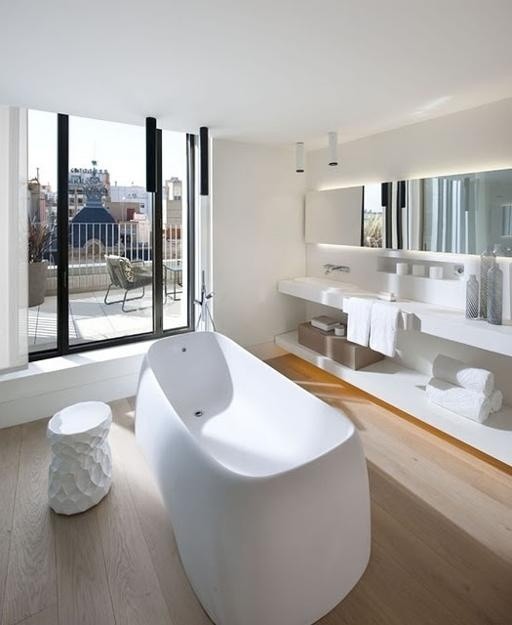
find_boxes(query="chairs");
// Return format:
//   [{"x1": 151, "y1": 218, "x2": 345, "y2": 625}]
[{"x1": 104, "y1": 254, "x2": 167, "y2": 313}]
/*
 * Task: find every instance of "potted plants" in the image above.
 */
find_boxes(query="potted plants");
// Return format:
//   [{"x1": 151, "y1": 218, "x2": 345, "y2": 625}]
[{"x1": 28, "y1": 212, "x2": 56, "y2": 308}]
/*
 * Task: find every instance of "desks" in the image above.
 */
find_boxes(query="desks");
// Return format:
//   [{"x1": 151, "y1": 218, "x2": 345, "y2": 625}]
[{"x1": 163, "y1": 260, "x2": 182, "y2": 301}]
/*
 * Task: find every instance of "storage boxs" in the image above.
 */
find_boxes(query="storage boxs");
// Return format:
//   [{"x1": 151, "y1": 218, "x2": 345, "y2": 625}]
[{"x1": 298, "y1": 322, "x2": 386, "y2": 370}]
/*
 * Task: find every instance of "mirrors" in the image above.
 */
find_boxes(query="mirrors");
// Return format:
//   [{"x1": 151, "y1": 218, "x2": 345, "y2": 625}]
[{"x1": 302, "y1": 167, "x2": 512, "y2": 258}]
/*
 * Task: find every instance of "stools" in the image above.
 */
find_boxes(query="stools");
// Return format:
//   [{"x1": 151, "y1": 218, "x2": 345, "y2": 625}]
[{"x1": 47, "y1": 401, "x2": 115, "y2": 516}]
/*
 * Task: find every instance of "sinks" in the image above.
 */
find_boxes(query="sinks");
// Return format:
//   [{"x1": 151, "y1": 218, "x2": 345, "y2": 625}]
[{"x1": 294, "y1": 276, "x2": 354, "y2": 289}]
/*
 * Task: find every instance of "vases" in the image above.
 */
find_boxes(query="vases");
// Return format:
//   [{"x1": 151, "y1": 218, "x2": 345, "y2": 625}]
[{"x1": 465, "y1": 248, "x2": 504, "y2": 325}]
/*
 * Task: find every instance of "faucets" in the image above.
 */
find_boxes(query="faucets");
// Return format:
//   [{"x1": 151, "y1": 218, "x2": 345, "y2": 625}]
[
  {"x1": 193, "y1": 270, "x2": 215, "y2": 334},
  {"x1": 324, "y1": 262, "x2": 351, "y2": 276}
]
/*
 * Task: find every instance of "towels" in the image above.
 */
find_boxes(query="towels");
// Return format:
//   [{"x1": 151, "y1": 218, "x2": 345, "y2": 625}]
[
  {"x1": 426, "y1": 354, "x2": 503, "y2": 423},
  {"x1": 346, "y1": 296, "x2": 400, "y2": 359}
]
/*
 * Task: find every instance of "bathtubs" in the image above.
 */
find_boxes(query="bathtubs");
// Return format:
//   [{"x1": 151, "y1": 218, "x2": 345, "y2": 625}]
[{"x1": 133, "y1": 327, "x2": 371, "y2": 625}]
